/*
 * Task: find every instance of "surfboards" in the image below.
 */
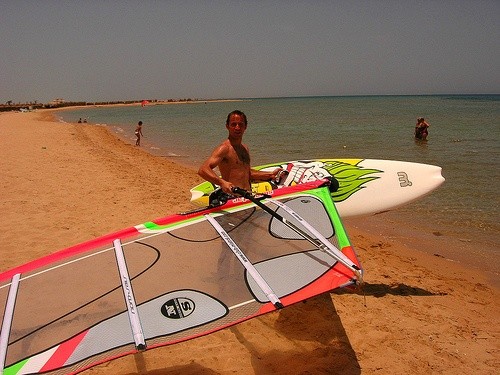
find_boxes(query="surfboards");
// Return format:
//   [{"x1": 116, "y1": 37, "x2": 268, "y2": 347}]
[{"x1": 189, "y1": 158, "x2": 446, "y2": 218}]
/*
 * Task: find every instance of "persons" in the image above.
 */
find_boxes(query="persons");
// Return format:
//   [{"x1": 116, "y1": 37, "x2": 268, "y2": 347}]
[
  {"x1": 415, "y1": 118, "x2": 429, "y2": 141},
  {"x1": 197, "y1": 110, "x2": 286, "y2": 298},
  {"x1": 134, "y1": 121, "x2": 144, "y2": 146}
]
